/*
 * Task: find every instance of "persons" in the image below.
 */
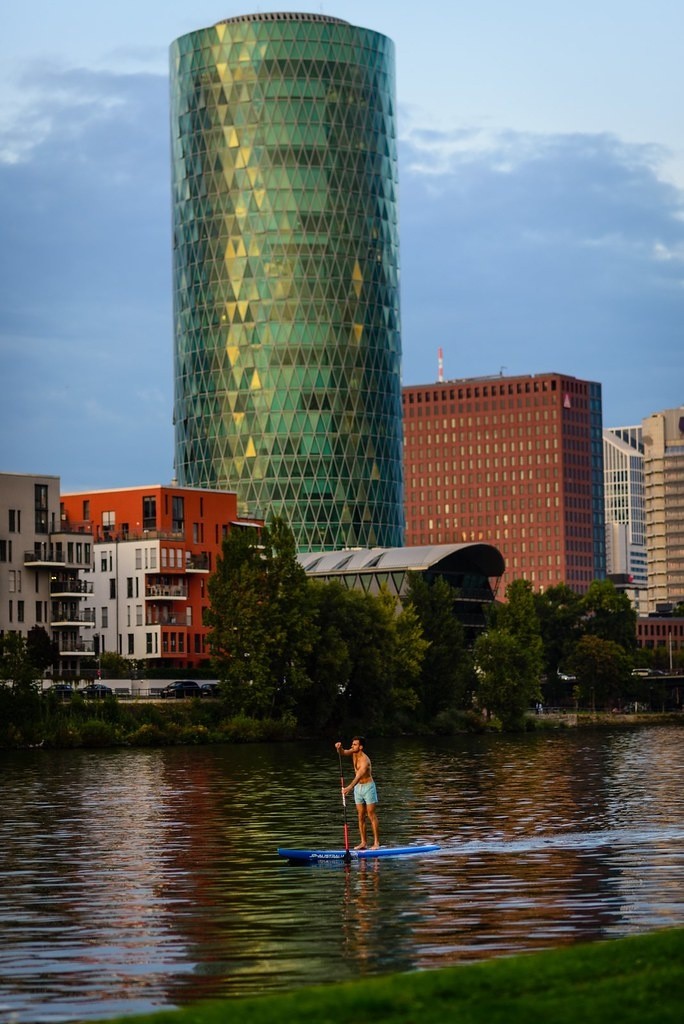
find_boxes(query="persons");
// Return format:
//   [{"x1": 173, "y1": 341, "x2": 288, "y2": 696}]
[{"x1": 336, "y1": 736, "x2": 379, "y2": 851}]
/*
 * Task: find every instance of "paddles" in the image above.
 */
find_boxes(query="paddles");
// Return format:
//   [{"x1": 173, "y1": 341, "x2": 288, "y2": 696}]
[{"x1": 337, "y1": 748, "x2": 353, "y2": 861}]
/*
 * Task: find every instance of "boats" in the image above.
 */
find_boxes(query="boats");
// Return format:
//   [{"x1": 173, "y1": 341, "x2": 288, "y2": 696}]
[{"x1": 276, "y1": 844, "x2": 442, "y2": 859}]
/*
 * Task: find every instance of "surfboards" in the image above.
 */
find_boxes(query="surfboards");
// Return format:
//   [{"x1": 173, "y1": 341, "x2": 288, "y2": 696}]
[{"x1": 277, "y1": 844, "x2": 441, "y2": 859}]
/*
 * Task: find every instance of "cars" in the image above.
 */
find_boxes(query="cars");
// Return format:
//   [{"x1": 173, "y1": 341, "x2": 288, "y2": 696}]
[
  {"x1": 43, "y1": 684, "x2": 74, "y2": 700},
  {"x1": 78, "y1": 684, "x2": 115, "y2": 698},
  {"x1": 630, "y1": 669, "x2": 679, "y2": 677},
  {"x1": 161, "y1": 681, "x2": 202, "y2": 699},
  {"x1": 200, "y1": 684, "x2": 221, "y2": 695}
]
